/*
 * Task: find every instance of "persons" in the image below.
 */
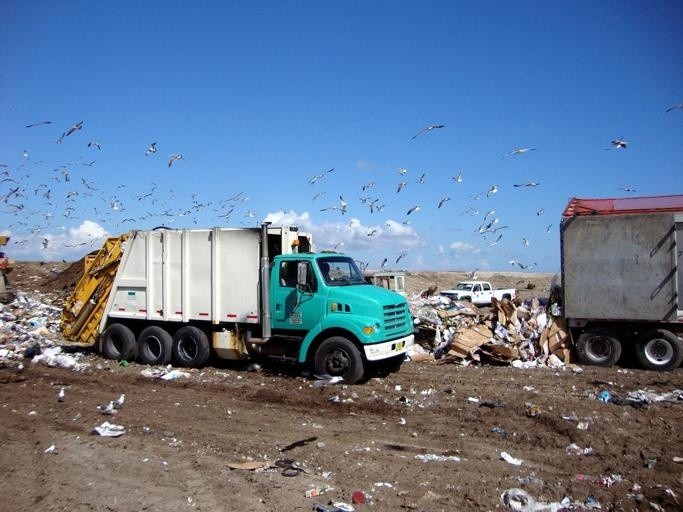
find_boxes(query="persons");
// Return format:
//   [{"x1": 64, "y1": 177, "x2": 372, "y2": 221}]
[
  {"x1": 313, "y1": 263, "x2": 330, "y2": 290},
  {"x1": 0, "y1": 252, "x2": 12, "y2": 289}
]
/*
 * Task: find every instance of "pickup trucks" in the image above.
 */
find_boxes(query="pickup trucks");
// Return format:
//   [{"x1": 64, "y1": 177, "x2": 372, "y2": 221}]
[{"x1": 440, "y1": 280, "x2": 520, "y2": 306}]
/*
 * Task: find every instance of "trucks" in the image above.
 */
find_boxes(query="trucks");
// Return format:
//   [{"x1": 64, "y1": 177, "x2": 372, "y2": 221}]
[
  {"x1": 561, "y1": 194, "x2": 682, "y2": 372},
  {"x1": 59, "y1": 222, "x2": 415, "y2": 386}
]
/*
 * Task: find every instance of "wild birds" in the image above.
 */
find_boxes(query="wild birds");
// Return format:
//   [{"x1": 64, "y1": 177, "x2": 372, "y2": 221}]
[
  {"x1": 0, "y1": 119, "x2": 639, "y2": 282},
  {"x1": 57, "y1": 387, "x2": 67, "y2": 401},
  {"x1": 96, "y1": 400, "x2": 115, "y2": 415},
  {"x1": 666, "y1": 104, "x2": 683, "y2": 113},
  {"x1": 113, "y1": 393, "x2": 125, "y2": 409},
  {"x1": 15, "y1": 361, "x2": 25, "y2": 373}
]
